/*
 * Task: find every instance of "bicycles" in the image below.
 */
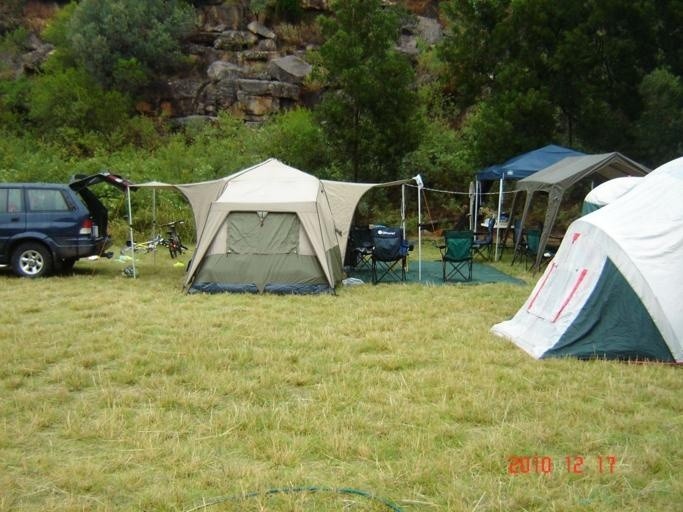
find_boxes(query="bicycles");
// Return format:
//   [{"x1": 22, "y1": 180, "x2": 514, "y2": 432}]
[{"x1": 161, "y1": 221, "x2": 185, "y2": 259}]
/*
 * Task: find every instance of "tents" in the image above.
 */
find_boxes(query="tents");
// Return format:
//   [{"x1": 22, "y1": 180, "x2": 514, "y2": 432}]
[
  {"x1": 468, "y1": 144, "x2": 654, "y2": 277},
  {"x1": 124, "y1": 154, "x2": 419, "y2": 297},
  {"x1": 488, "y1": 158, "x2": 683, "y2": 364}
]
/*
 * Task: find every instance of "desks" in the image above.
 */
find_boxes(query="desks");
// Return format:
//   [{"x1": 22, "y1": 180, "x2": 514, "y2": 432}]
[{"x1": 480, "y1": 222, "x2": 526, "y2": 248}]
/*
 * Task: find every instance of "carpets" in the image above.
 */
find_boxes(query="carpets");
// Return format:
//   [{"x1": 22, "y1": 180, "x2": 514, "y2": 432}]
[{"x1": 339, "y1": 260, "x2": 528, "y2": 284}]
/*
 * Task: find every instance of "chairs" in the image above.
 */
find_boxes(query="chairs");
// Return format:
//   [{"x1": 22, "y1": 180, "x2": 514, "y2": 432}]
[
  {"x1": 371, "y1": 228, "x2": 409, "y2": 282},
  {"x1": 512, "y1": 220, "x2": 524, "y2": 265},
  {"x1": 524, "y1": 230, "x2": 542, "y2": 273},
  {"x1": 471, "y1": 217, "x2": 495, "y2": 261},
  {"x1": 441, "y1": 231, "x2": 472, "y2": 284},
  {"x1": 352, "y1": 229, "x2": 372, "y2": 275}
]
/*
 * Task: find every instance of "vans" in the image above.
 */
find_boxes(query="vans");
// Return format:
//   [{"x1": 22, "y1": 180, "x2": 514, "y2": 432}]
[{"x1": 0, "y1": 172, "x2": 138, "y2": 278}]
